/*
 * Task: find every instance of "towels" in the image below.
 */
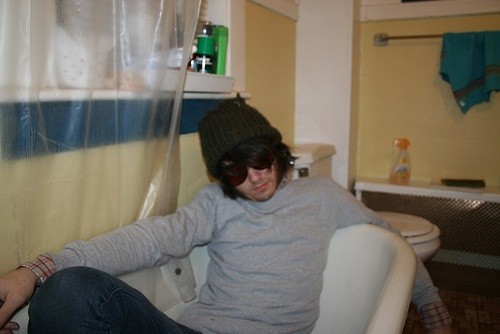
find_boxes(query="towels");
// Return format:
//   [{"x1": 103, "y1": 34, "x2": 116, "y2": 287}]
[{"x1": 439, "y1": 29, "x2": 500, "y2": 114}]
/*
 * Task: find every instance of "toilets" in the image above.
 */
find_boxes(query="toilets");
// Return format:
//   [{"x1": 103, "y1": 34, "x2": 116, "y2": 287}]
[{"x1": 280, "y1": 143, "x2": 442, "y2": 262}]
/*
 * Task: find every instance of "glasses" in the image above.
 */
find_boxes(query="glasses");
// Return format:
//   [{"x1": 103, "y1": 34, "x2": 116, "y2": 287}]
[{"x1": 221, "y1": 145, "x2": 273, "y2": 187}]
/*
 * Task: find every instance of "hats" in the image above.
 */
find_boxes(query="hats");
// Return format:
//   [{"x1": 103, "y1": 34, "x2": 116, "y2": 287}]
[{"x1": 199, "y1": 101, "x2": 281, "y2": 177}]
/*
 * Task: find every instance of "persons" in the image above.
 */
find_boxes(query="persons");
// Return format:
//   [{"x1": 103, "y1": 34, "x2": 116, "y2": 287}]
[{"x1": 0, "y1": 97, "x2": 454, "y2": 334}]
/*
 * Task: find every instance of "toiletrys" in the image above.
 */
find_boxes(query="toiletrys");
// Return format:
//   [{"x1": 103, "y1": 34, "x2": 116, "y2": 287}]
[
  {"x1": 212, "y1": 24, "x2": 228, "y2": 74},
  {"x1": 194, "y1": 24, "x2": 215, "y2": 75}
]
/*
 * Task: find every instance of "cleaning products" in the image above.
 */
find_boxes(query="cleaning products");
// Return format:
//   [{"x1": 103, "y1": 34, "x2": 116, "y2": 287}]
[{"x1": 389, "y1": 138, "x2": 412, "y2": 185}]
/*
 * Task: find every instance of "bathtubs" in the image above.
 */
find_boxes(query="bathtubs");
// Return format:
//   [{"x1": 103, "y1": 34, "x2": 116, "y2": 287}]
[{"x1": 9, "y1": 221, "x2": 418, "y2": 334}]
